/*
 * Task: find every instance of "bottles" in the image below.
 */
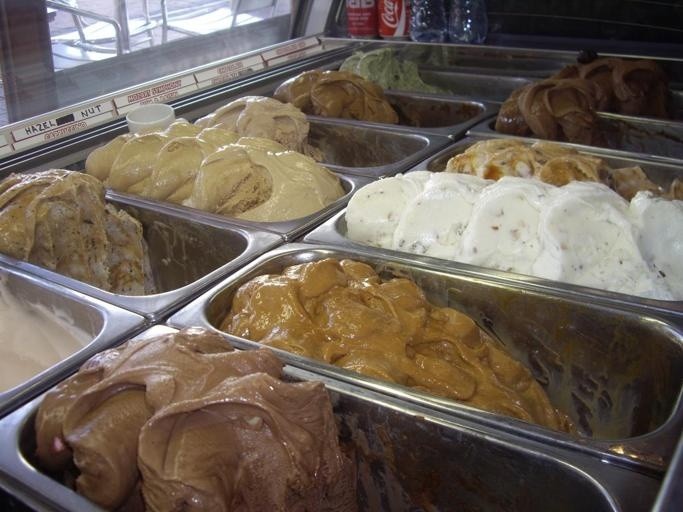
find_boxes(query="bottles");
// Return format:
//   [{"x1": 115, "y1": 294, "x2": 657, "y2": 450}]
[
  {"x1": 446, "y1": 0, "x2": 487, "y2": 45},
  {"x1": 410, "y1": 0, "x2": 448, "y2": 44}
]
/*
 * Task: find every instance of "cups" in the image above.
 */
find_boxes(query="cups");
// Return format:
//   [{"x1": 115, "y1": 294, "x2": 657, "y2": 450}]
[{"x1": 125, "y1": 103, "x2": 175, "y2": 131}]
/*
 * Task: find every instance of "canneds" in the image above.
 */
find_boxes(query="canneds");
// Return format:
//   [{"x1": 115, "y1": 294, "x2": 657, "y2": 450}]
[
  {"x1": 377, "y1": 0, "x2": 410, "y2": 40},
  {"x1": 345, "y1": 0, "x2": 377, "y2": 40}
]
